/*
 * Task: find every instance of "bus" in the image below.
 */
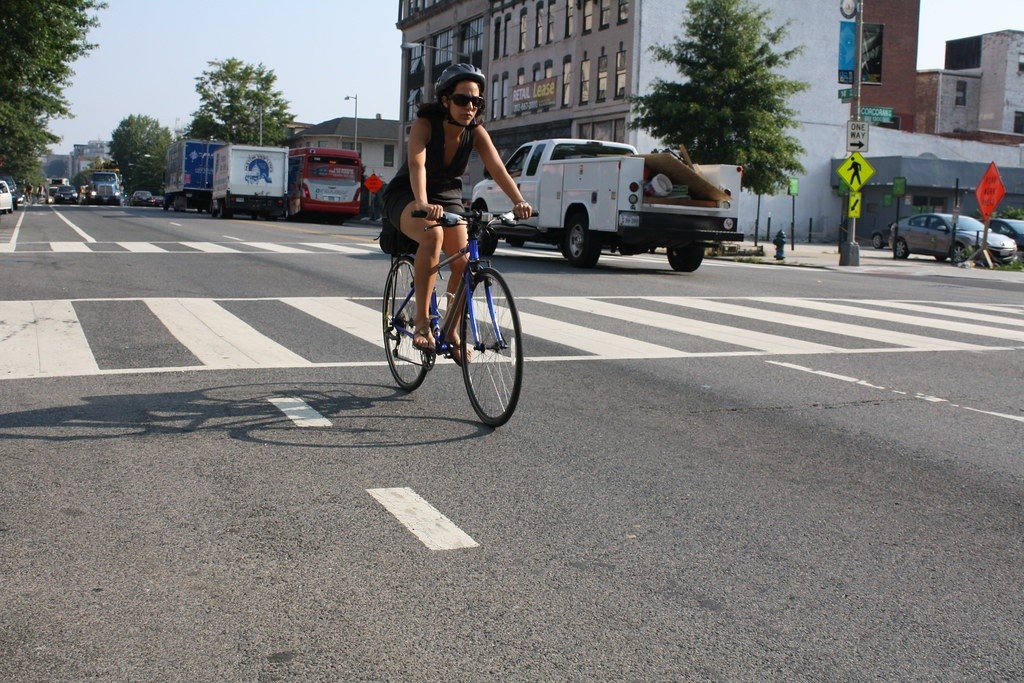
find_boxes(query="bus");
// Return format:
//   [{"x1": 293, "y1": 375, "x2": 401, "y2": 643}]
[{"x1": 280, "y1": 146, "x2": 363, "y2": 224}]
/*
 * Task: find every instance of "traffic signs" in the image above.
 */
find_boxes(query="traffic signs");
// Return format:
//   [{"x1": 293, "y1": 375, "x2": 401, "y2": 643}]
[{"x1": 845, "y1": 120, "x2": 869, "y2": 153}]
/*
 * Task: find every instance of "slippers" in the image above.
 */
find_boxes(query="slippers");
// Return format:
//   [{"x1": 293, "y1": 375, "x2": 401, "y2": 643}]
[
  {"x1": 449, "y1": 340, "x2": 473, "y2": 368},
  {"x1": 412, "y1": 325, "x2": 436, "y2": 352}
]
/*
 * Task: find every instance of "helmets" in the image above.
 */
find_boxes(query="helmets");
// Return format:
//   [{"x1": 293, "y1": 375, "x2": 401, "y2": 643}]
[{"x1": 436, "y1": 63, "x2": 485, "y2": 98}]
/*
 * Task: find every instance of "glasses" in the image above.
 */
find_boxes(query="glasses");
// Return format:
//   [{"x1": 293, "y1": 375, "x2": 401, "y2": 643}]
[{"x1": 448, "y1": 93, "x2": 484, "y2": 107}]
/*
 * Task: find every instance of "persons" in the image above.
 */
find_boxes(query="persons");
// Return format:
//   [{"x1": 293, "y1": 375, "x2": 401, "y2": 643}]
[
  {"x1": 26, "y1": 182, "x2": 33, "y2": 202},
  {"x1": 381, "y1": 64, "x2": 533, "y2": 367},
  {"x1": 36, "y1": 183, "x2": 44, "y2": 203}
]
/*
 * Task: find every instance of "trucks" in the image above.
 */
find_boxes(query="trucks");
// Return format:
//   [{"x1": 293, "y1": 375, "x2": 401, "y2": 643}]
[
  {"x1": 206, "y1": 144, "x2": 290, "y2": 222},
  {"x1": 85, "y1": 156, "x2": 125, "y2": 205},
  {"x1": 470, "y1": 137, "x2": 743, "y2": 273},
  {"x1": 162, "y1": 138, "x2": 227, "y2": 213}
]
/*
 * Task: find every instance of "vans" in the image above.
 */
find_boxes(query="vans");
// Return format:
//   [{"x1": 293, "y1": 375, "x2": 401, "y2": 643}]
[{"x1": 0, "y1": 175, "x2": 20, "y2": 210}]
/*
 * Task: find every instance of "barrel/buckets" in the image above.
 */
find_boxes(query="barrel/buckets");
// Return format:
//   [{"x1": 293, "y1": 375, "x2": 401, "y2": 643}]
[{"x1": 644, "y1": 174, "x2": 673, "y2": 197}]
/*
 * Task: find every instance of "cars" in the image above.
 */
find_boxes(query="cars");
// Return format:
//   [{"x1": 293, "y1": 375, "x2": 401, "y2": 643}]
[
  {"x1": 37, "y1": 177, "x2": 87, "y2": 205},
  {"x1": 129, "y1": 191, "x2": 153, "y2": 207},
  {"x1": 0, "y1": 181, "x2": 14, "y2": 214},
  {"x1": 975, "y1": 218, "x2": 1024, "y2": 252},
  {"x1": 871, "y1": 216, "x2": 911, "y2": 249},
  {"x1": 888, "y1": 212, "x2": 1019, "y2": 264}
]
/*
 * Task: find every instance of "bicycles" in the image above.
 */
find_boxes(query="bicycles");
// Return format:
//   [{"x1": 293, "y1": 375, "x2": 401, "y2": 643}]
[{"x1": 381, "y1": 207, "x2": 542, "y2": 427}]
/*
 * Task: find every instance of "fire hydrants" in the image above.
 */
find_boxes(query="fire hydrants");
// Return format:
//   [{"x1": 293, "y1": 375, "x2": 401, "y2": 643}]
[{"x1": 772, "y1": 229, "x2": 787, "y2": 260}]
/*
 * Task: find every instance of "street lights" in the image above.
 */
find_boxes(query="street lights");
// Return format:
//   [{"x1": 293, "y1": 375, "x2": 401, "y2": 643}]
[
  {"x1": 400, "y1": 42, "x2": 473, "y2": 65},
  {"x1": 219, "y1": 101, "x2": 264, "y2": 147},
  {"x1": 345, "y1": 94, "x2": 358, "y2": 150}
]
